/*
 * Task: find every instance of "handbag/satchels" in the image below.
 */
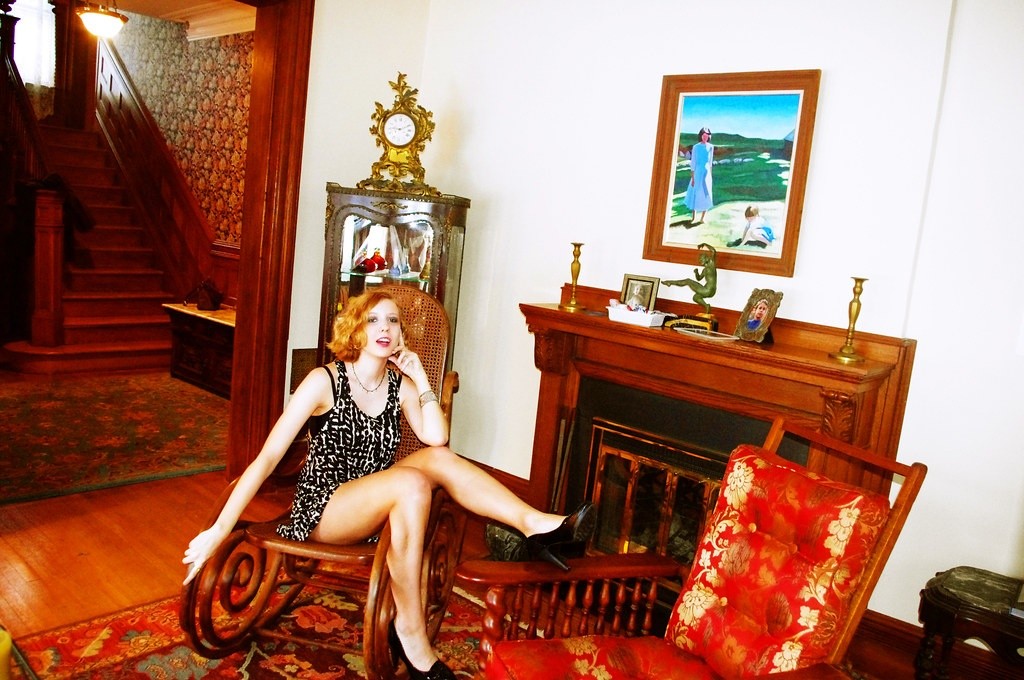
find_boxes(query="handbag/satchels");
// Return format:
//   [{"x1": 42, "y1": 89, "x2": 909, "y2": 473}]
[{"x1": 183, "y1": 280, "x2": 220, "y2": 310}]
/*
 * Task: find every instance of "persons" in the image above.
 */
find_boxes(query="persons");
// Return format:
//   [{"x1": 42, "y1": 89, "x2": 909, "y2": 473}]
[
  {"x1": 747, "y1": 300, "x2": 768, "y2": 329},
  {"x1": 183, "y1": 293, "x2": 597, "y2": 680},
  {"x1": 661, "y1": 243, "x2": 718, "y2": 313},
  {"x1": 628, "y1": 284, "x2": 646, "y2": 306}
]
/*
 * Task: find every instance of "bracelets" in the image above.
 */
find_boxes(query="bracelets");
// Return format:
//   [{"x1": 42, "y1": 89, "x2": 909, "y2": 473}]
[{"x1": 420, "y1": 390, "x2": 439, "y2": 407}]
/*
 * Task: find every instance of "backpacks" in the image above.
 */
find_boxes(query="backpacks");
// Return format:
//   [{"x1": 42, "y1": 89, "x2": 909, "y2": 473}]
[{"x1": 757, "y1": 227, "x2": 775, "y2": 242}]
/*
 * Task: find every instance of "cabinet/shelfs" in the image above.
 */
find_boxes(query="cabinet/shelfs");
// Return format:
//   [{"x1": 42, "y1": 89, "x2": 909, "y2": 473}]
[
  {"x1": 162, "y1": 300, "x2": 243, "y2": 400},
  {"x1": 320, "y1": 184, "x2": 468, "y2": 442}
]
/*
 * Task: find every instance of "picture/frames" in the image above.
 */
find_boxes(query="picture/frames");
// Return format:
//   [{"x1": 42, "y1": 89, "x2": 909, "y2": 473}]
[
  {"x1": 619, "y1": 273, "x2": 660, "y2": 312},
  {"x1": 733, "y1": 288, "x2": 783, "y2": 342},
  {"x1": 642, "y1": 68, "x2": 821, "y2": 278}
]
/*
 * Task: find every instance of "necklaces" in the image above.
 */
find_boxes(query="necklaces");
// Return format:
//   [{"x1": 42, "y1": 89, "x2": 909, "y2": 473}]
[{"x1": 352, "y1": 362, "x2": 385, "y2": 392}]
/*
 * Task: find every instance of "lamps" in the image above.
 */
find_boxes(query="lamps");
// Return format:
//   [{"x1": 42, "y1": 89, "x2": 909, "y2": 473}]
[{"x1": 75, "y1": 0, "x2": 129, "y2": 35}]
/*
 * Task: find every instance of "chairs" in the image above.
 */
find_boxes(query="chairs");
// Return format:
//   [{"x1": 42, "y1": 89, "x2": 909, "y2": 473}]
[
  {"x1": 458, "y1": 414, "x2": 927, "y2": 680},
  {"x1": 179, "y1": 284, "x2": 468, "y2": 680}
]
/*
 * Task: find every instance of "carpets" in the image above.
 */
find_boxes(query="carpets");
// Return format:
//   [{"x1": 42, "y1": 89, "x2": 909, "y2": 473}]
[
  {"x1": 1, "y1": 539, "x2": 552, "y2": 679},
  {"x1": 0, "y1": 365, "x2": 239, "y2": 506}
]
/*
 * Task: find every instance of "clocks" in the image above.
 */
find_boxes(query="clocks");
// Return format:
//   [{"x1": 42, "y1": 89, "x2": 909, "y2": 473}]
[{"x1": 356, "y1": 67, "x2": 442, "y2": 197}]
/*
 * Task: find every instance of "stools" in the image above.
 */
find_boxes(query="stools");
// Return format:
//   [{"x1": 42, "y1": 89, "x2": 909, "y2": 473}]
[{"x1": 918, "y1": 566, "x2": 1024, "y2": 680}]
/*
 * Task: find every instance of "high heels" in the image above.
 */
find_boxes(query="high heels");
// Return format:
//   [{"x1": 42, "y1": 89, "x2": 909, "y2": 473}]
[
  {"x1": 527, "y1": 501, "x2": 596, "y2": 570},
  {"x1": 387, "y1": 618, "x2": 456, "y2": 680}
]
